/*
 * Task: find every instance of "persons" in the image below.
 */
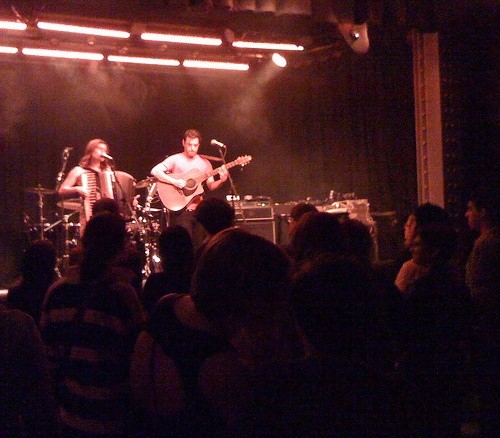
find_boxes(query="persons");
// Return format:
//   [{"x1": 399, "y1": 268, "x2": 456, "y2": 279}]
[
  {"x1": 57, "y1": 139, "x2": 116, "y2": 239},
  {"x1": 150, "y1": 129, "x2": 230, "y2": 240},
  {"x1": 0, "y1": 188, "x2": 500, "y2": 438}
]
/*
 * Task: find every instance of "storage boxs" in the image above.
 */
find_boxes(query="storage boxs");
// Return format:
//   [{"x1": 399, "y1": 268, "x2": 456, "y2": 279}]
[
  {"x1": 234, "y1": 222, "x2": 275, "y2": 243},
  {"x1": 233, "y1": 206, "x2": 274, "y2": 220},
  {"x1": 368, "y1": 212, "x2": 400, "y2": 268}
]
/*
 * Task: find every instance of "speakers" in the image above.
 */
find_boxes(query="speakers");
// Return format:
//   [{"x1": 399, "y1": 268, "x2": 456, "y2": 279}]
[{"x1": 232, "y1": 205, "x2": 294, "y2": 246}]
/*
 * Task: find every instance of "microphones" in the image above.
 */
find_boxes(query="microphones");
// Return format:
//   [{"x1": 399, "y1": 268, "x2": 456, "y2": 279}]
[
  {"x1": 210, "y1": 139, "x2": 226, "y2": 148},
  {"x1": 97, "y1": 151, "x2": 113, "y2": 160}
]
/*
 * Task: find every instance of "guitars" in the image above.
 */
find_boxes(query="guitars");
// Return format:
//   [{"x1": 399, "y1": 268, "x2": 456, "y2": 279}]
[{"x1": 156, "y1": 155, "x2": 252, "y2": 212}]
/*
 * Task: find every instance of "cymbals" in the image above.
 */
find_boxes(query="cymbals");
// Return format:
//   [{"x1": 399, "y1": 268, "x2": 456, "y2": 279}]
[
  {"x1": 56, "y1": 198, "x2": 82, "y2": 210},
  {"x1": 22, "y1": 187, "x2": 55, "y2": 195},
  {"x1": 135, "y1": 177, "x2": 155, "y2": 189}
]
